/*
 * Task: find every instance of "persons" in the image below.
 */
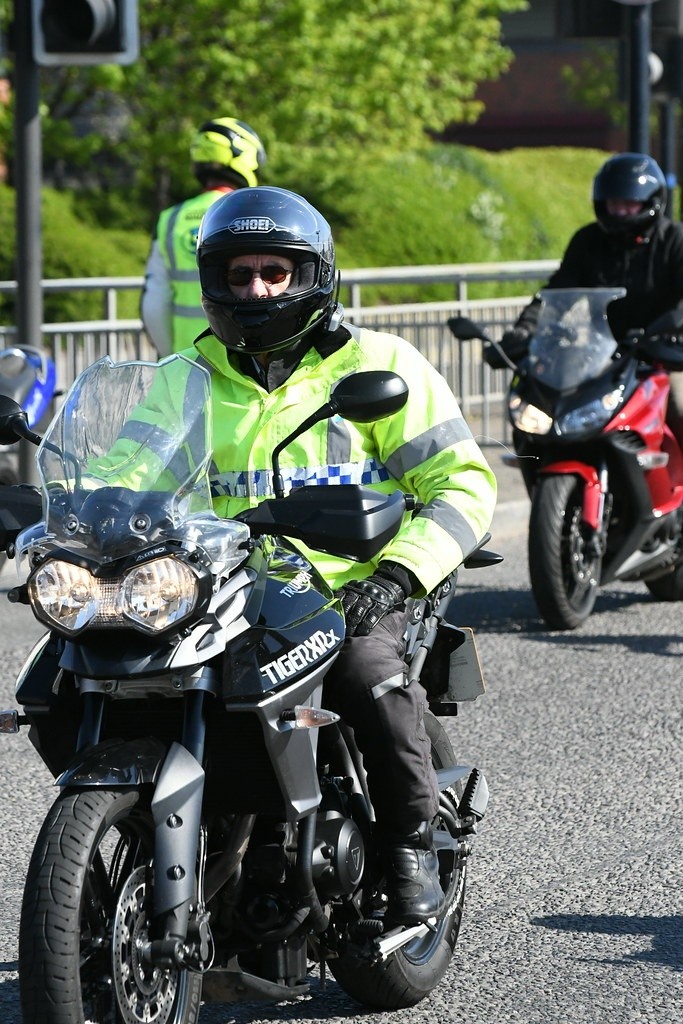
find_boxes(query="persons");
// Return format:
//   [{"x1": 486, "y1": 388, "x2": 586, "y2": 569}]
[
  {"x1": 1, "y1": 187, "x2": 498, "y2": 918},
  {"x1": 483, "y1": 154, "x2": 683, "y2": 451},
  {"x1": 139, "y1": 116, "x2": 266, "y2": 360}
]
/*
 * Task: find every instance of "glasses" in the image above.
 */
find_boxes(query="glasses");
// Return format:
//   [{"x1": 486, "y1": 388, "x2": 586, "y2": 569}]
[{"x1": 224, "y1": 265, "x2": 295, "y2": 287}]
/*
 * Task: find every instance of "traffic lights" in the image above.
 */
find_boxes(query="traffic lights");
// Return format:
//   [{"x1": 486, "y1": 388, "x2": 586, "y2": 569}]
[{"x1": 33, "y1": 0, "x2": 142, "y2": 69}]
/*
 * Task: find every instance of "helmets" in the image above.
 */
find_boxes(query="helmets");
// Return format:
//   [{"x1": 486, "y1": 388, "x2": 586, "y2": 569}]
[
  {"x1": 593, "y1": 152, "x2": 668, "y2": 242},
  {"x1": 196, "y1": 186, "x2": 344, "y2": 355},
  {"x1": 189, "y1": 117, "x2": 265, "y2": 187}
]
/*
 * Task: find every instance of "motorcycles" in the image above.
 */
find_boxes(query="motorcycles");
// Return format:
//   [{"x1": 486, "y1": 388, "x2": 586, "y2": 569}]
[
  {"x1": 0, "y1": 342, "x2": 506, "y2": 1023},
  {"x1": 446, "y1": 284, "x2": 683, "y2": 629}
]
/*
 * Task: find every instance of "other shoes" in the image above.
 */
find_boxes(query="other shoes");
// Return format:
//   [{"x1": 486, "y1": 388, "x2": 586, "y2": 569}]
[{"x1": 382, "y1": 822, "x2": 447, "y2": 924}]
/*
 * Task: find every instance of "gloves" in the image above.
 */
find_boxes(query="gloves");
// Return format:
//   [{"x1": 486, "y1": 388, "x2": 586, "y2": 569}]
[
  {"x1": 333, "y1": 575, "x2": 406, "y2": 637},
  {"x1": 486, "y1": 330, "x2": 527, "y2": 368}
]
